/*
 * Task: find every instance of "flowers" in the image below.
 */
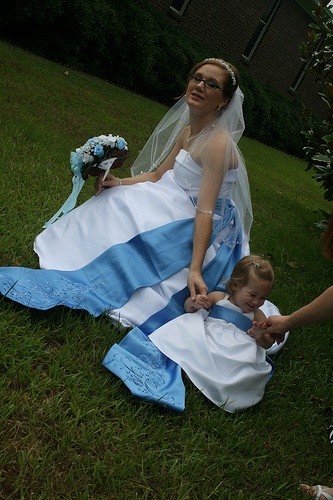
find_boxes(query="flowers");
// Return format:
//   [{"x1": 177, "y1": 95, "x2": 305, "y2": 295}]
[{"x1": 42, "y1": 134, "x2": 129, "y2": 228}]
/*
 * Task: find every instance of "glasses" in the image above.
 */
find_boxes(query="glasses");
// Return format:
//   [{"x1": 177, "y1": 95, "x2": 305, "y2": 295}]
[{"x1": 189, "y1": 73, "x2": 225, "y2": 93}]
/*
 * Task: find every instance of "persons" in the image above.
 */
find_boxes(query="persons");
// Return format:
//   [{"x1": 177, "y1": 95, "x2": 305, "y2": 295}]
[
  {"x1": 183, "y1": 255, "x2": 289, "y2": 413},
  {"x1": 251, "y1": 214, "x2": 333, "y2": 500},
  {"x1": 0, "y1": 57, "x2": 252, "y2": 411}
]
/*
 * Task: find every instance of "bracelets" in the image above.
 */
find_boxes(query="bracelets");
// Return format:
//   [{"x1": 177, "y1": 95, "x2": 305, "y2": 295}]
[{"x1": 116, "y1": 178, "x2": 122, "y2": 186}]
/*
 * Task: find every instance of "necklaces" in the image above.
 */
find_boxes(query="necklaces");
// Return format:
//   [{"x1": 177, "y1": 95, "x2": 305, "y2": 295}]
[{"x1": 186, "y1": 120, "x2": 216, "y2": 144}]
